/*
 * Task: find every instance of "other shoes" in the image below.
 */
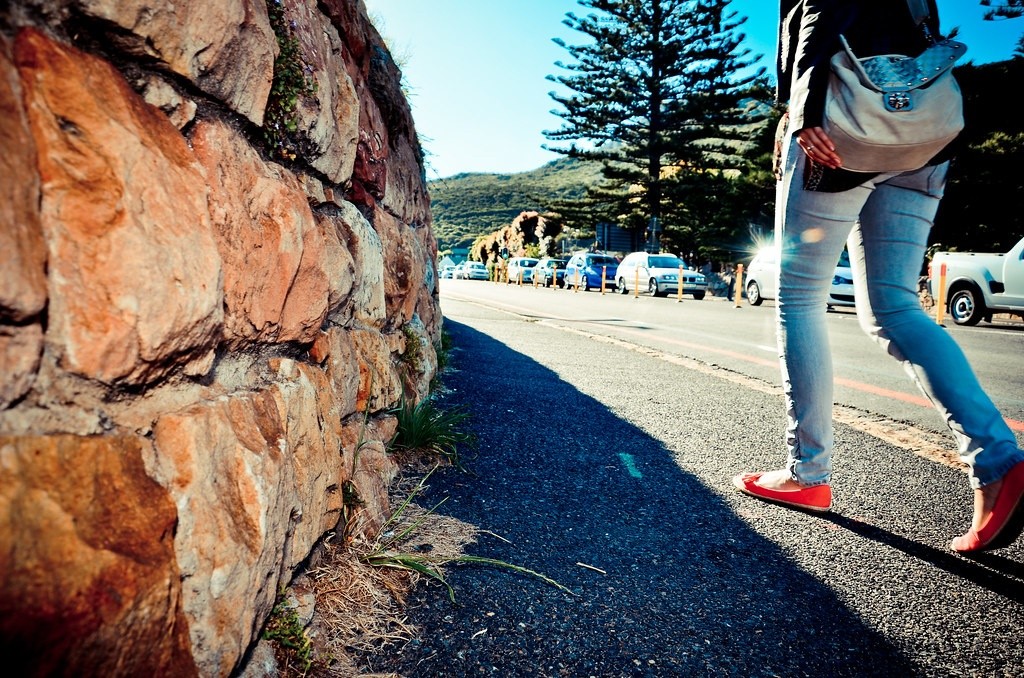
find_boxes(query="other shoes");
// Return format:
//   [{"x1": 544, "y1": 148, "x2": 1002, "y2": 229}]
[
  {"x1": 732, "y1": 473, "x2": 833, "y2": 512},
  {"x1": 951, "y1": 458, "x2": 1024, "y2": 553}
]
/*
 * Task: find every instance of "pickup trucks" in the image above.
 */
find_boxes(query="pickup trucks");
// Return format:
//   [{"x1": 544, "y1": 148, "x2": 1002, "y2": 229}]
[{"x1": 931, "y1": 236, "x2": 1024, "y2": 326}]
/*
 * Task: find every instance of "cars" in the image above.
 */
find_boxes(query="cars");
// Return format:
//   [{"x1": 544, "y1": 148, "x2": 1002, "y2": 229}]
[
  {"x1": 507, "y1": 257, "x2": 539, "y2": 286},
  {"x1": 462, "y1": 261, "x2": 490, "y2": 281},
  {"x1": 615, "y1": 251, "x2": 708, "y2": 301},
  {"x1": 453, "y1": 264, "x2": 464, "y2": 279},
  {"x1": 529, "y1": 258, "x2": 568, "y2": 288},
  {"x1": 563, "y1": 253, "x2": 620, "y2": 291},
  {"x1": 745, "y1": 244, "x2": 856, "y2": 313},
  {"x1": 441, "y1": 265, "x2": 456, "y2": 279}
]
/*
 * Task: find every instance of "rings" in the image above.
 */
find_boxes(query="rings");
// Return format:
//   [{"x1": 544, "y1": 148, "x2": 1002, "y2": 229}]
[{"x1": 807, "y1": 146, "x2": 815, "y2": 150}]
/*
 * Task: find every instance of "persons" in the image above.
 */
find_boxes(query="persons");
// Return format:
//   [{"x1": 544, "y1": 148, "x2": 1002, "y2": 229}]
[{"x1": 730, "y1": 0, "x2": 1023, "y2": 554}]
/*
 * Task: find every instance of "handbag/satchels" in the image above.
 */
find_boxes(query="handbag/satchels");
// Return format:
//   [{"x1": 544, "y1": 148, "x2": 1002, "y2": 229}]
[{"x1": 819, "y1": 36, "x2": 969, "y2": 174}]
